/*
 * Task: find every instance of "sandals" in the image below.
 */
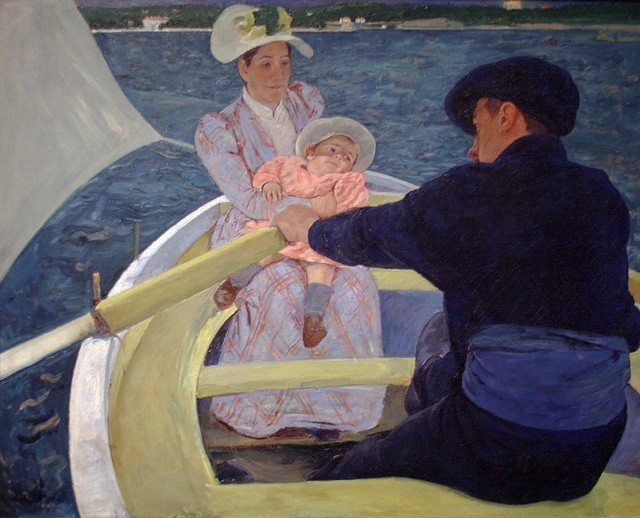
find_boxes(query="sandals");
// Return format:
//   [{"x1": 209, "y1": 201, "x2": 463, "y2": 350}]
[
  {"x1": 303, "y1": 317, "x2": 327, "y2": 349},
  {"x1": 214, "y1": 278, "x2": 241, "y2": 309}
]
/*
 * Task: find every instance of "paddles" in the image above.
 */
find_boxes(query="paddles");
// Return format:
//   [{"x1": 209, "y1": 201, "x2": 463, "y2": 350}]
[{"x1": 0, "y1": 224, "x2": 293, "y2": 386}]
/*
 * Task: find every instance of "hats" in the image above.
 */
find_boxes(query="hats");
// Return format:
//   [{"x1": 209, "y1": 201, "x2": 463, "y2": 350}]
[
  {"x1": 295, "y1": 117, "x2": 375, "y2": 172},
  {"x1": 444, "y1": 56, "x2": 579, "y2": 136},
  {"x1": 210, "y1": 4, "x2": 314, "y2": 63}
]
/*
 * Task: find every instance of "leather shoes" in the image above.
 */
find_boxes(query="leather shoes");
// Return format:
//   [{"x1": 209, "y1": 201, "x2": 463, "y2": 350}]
[{"x1": 405, "y1": 311, "x2": 452, "y2": 416}]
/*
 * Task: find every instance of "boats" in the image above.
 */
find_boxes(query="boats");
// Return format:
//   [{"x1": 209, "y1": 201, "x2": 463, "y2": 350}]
[{"x1": 68, "y1": 170, "x2": 640, "y2": 517}]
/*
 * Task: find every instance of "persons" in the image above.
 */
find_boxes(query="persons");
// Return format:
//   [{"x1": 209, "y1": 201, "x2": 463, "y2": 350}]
[
  {"x1": 195, "y1": 4, "x2": 390, "y2": 440},
  {"x1": 215, "y1": 113, "x2": 379, "y2": 349},
  {"x1": 270, "y1": 54, "x2": 639, "y2": 506}
]
